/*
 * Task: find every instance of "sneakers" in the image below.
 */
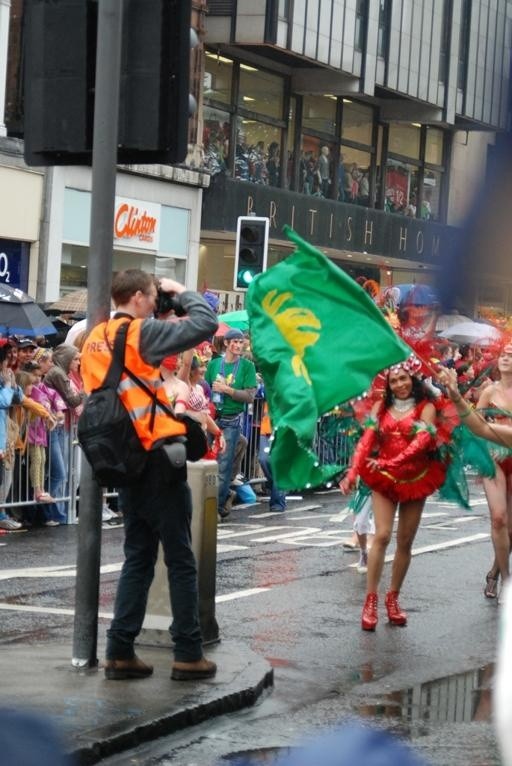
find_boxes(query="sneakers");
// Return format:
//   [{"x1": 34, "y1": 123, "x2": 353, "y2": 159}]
[{"x1": 0, "y1": 505, "x2": 118, "y2": 529}]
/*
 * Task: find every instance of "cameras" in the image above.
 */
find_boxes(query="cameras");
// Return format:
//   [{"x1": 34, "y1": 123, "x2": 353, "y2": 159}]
[{"x1": 154, "y1": 288, "x2": 187, "y2": 318}]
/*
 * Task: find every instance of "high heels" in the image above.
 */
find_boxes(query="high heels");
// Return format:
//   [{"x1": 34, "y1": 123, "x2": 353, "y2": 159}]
[
  {"x1": 384, "y1": 592, "x2": 407, "y2": 624},
  {"x1": 360, "y1": 592, "x2": 378, "y2": 630}
]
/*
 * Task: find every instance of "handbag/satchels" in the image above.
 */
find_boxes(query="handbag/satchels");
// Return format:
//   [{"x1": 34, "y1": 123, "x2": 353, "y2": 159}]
[
  {"x1": 77, "y1": 386, "x2": 148, "y2": 491},
  {"x1": 177, "y1": 413, "x2": 209, "y2": 462}
]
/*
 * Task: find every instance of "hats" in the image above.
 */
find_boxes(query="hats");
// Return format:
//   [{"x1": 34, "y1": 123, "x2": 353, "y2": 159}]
[
  {"x1": 16, "y1": 337, "x2": 38, "y2": 350},
  {"x1": 223, "y1": 327, "x2": 244, "y2": 339}
]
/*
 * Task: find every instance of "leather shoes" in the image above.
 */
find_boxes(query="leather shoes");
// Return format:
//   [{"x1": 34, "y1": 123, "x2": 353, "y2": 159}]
[
  {"x1": 169, "y1": 656, "x2": 217, "y2": 680},
  {"x1": 344, "y1": 542, "x2": 362, "y2": 549},
  {"x1": 105, "y1": 655, "x2": 154, "y2": 680},
  {"x1": 484, "y1": 570, "x2": 498, "y2": 599},
  {"x1": 356, "y1": 552, "x2": 368, "y2": 575},
  {"x1": 218, "y1": 489, "x2": 236, "y2": 518}
]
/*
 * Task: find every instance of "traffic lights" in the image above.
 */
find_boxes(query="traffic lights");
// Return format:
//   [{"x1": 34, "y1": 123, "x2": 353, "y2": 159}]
[{"x1": 232, "y1": 215, "x2": 271, "y2": 291}]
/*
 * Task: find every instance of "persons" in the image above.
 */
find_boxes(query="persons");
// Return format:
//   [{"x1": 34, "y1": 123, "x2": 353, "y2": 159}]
[
  {"x1": 82, "y1": 269, "x2": 220, "y2": 683},
  {"x1": 338, "y1": 318, "x2": 512, "y2": 630},
  {"x1": 4, "y1": 308, "x2": 338, "y2": 529},
  {"x1": 203, "y1": 119, "x2": 432, "y2": 220}
]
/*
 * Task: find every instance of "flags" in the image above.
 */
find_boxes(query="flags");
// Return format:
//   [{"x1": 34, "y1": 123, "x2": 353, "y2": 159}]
[{"x1": 245, "y1": 225, "x2": 413, "y2": 491}]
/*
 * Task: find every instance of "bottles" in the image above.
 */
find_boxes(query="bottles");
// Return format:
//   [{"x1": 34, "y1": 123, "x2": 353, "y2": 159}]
[{"x1": 210, "y1": 379, "x2": 222, "y2": 411}]
